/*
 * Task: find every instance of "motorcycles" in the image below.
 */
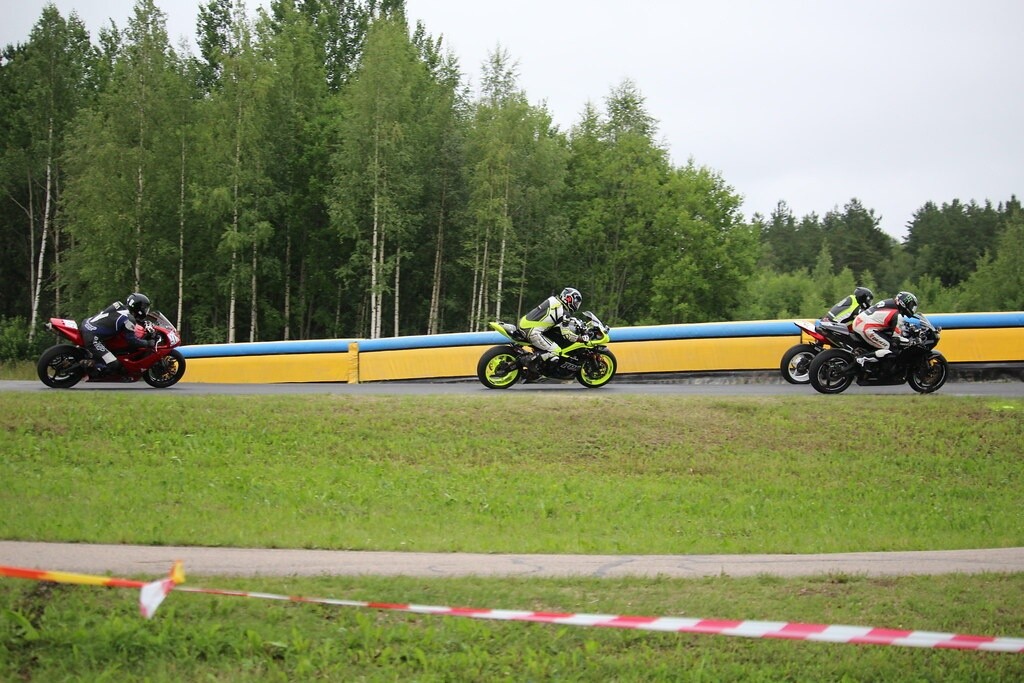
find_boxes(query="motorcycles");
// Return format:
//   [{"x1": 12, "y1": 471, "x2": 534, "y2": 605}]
[
  {"x1": 780, "y1": 311, "x2": 950, "y2": 395},
  {"x1": 36, "y1": 308, "x2": 186, "y2": 389},
  {"x1": 476, "y1": 310, "x2": 618, "y2": 390}
]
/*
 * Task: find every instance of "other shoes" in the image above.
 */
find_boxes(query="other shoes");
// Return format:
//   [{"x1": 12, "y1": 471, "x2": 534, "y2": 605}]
[{"x1": 80, "y1": 360, "x2": 100, "y2": 377}]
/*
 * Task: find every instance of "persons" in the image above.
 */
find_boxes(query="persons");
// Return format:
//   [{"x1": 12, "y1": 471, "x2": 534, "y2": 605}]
[
  {"x1": 78, "y1": 293, "x2": 156, "y2": 380},
  {"x1": 520, "y1": 287, "x2": 589, "y2": 375},
  {"x1": 815, "y1": 287, "x2": 874, "y2": 348},
  {"x1": 852, "y1": 292, "x2": 918, "y2": 375}
]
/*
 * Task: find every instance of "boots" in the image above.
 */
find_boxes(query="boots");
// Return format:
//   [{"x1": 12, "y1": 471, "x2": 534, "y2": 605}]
[
  {"x1": 854, "y1": 351, "x2": 880, "y2": 375},
  {"x1": 521, "y1": 352, "x2": 544, "y2": 375}
]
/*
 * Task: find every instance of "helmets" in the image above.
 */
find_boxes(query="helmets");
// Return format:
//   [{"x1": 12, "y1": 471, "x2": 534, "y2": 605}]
[
  {"x1": 560, "y1": 287, "x2": 582, "y2": 314},
  {"x1": 854, "y1": 287, "x2": 874, "y2": 311},
  {"x1": 127, "y1": 292, "x2": 151, "y2": 320},
  {"x1": 895, "y1": 292, "x2": 918, "y2": 318}
]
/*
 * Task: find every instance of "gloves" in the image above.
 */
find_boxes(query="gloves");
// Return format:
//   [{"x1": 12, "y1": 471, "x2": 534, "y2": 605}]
[
  {"x1": 580, "y1": 335, "x2": 589, "y2": 343},
  {"x1": 909, "y1": 338, "x2": 926, "y2": 349},
  {"x1": 147, "y1": 340, "x2": 155, "y2": 347}
]
